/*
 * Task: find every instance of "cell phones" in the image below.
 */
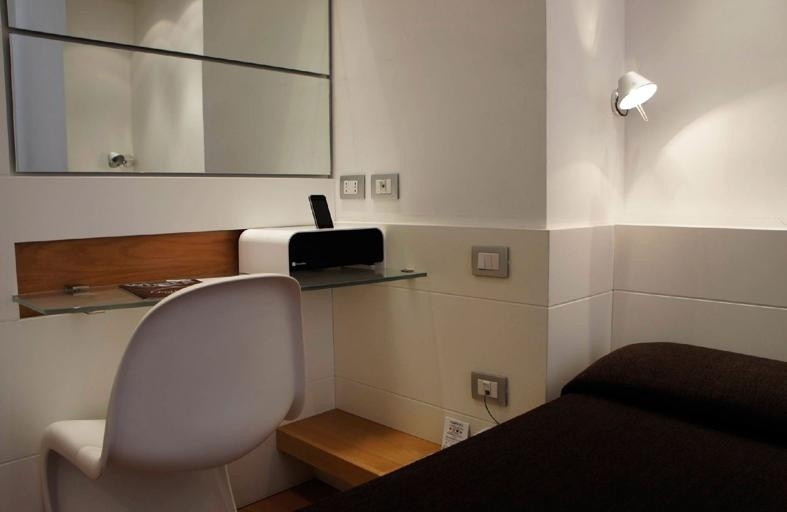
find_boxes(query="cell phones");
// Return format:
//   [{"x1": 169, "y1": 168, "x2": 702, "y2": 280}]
[{"x1": 309, "y1": 193, "x2": 334, "y2": 229}]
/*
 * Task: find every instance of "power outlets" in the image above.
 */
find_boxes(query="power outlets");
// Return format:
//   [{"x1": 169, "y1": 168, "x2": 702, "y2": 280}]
[
  {"x1": 340, "y1": 175, "x2": 365, "y2": 199},
  {"x1": 471, "y1": 370, "x2": 508, "y2": 407}
]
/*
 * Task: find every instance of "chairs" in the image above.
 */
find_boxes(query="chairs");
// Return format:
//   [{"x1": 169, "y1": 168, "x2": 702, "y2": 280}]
[{"x1": 39, "y1": 272, "x2": 307, "y2": 512}]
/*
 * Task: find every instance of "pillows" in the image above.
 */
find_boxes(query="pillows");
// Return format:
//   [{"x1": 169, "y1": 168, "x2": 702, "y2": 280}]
[{"x1": 561, "y1": 344, "x2": 787, "y2": 448}]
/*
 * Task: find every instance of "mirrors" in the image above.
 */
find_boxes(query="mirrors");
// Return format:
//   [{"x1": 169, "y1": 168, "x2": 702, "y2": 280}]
[{"x1": 0, "y1": 0, "x2": 333, "y2": 178}]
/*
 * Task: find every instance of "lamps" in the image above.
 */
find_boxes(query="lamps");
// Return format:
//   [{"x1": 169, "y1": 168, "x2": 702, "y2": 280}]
[
  {"x1": 108, "y1": 152, "x2": 134, "y2": 168},
  {"x1": 611, "y1": 71, "x2": 657, "y2": 121}
]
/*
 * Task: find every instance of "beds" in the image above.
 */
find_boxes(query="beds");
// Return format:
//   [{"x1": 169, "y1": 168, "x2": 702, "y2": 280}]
[{"x1": 292, "y1": 393, "x2": 787, "y2": 512}]
